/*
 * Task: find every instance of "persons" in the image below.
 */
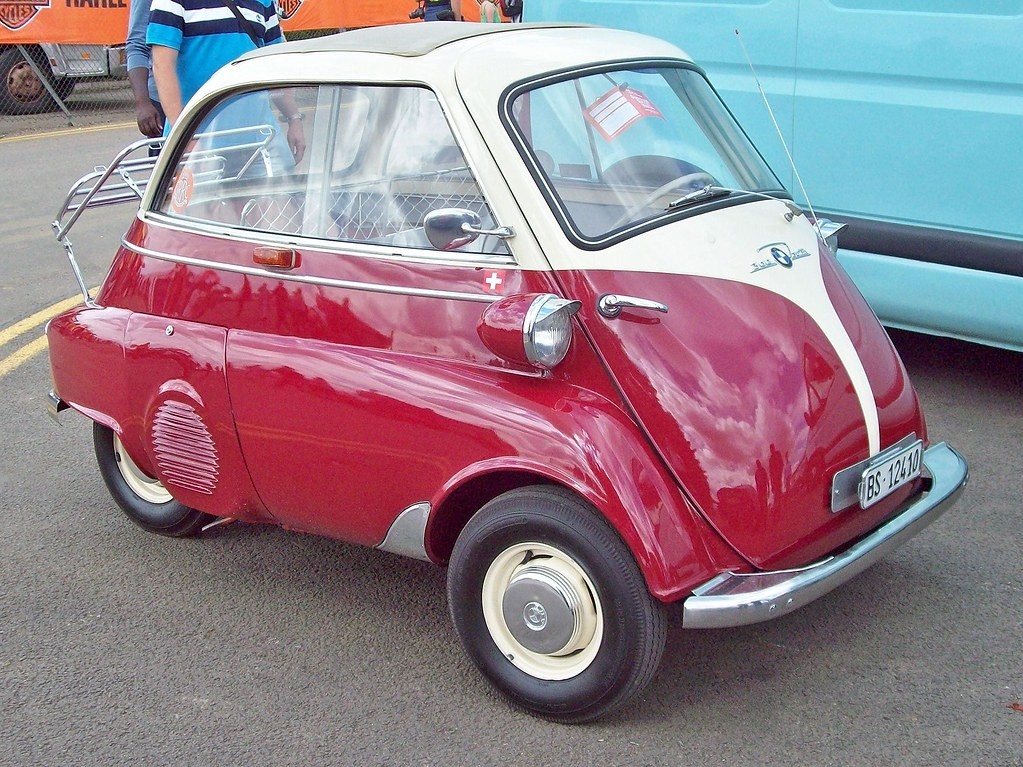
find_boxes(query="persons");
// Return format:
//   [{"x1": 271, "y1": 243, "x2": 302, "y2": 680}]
[
  {"x1": 144, "y1": 0, "x2": 311, "y2": 186},
  {"x1": 416, "y1": 0, "x2": 462, "y2": 21},
  {"x1": 124, "y1": 1, "x2": 165, "y2": 160},
  {"x1": 481, "y1": 0, "x2": 500, "y2": 23},
  {"x1": 510, "y1": 5, "x2": 522, "y2": 24}
]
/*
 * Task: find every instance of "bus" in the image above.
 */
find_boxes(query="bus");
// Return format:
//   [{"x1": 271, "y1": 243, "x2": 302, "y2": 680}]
[{"x1": 0, "y1": 1, "x2": 517, "y2": 122}]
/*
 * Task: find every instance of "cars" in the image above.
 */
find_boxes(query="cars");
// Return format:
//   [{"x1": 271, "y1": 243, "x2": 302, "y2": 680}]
[{"x1": 53, "y1": 21, "x2": 968, "y2": 725}]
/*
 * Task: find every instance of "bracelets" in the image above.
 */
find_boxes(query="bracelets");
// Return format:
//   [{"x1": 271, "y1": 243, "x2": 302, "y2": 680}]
[{"x1": 286, "y1": 112, "x2": 306, "y2": 123}]
